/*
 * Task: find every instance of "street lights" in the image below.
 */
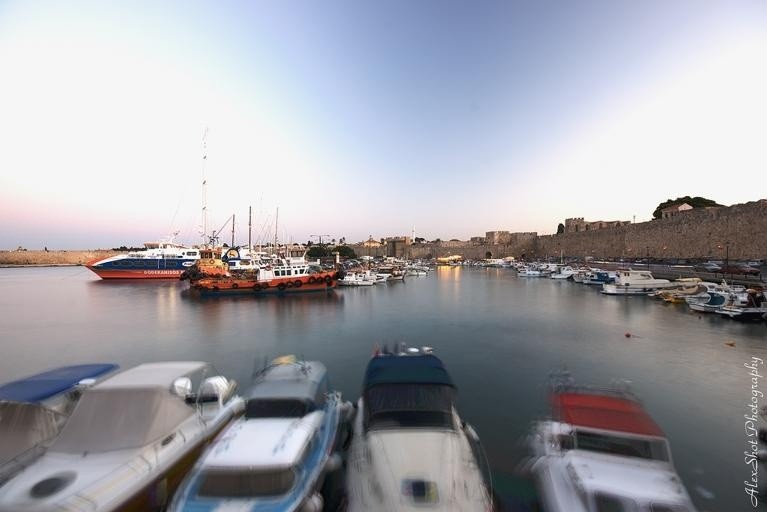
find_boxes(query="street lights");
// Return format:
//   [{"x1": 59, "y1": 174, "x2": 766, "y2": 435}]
[{"x1": 717, "y1": 245, "x2": 723, "y2": 259}]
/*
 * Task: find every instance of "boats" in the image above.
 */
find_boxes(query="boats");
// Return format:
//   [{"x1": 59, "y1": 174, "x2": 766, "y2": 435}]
[
  {"x1": 339, "y1": 345, "x2": 494, "y2": 512},
  {"x1": 529, "y1": 383, "x2": 699, "y2": 512},
  {"x1": 0, "y1": 363, "x2": 120, "y2": 485},
  {"x1": 164, "y1": 354, "x2": 354, "y2": 511},
  {"x1": 0, "y1": 359, "x2": 244, "y2": 511}
]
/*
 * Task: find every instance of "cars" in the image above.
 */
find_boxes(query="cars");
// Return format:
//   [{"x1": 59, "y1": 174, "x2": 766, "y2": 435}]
[
  {"x1": 720, "y1": 263, "x2": 760, "y2": 275},
  {"x1": 748, "y1": 262, "x2": 760, "y2": 268},
  {"x1": 691, "y1": 263, "x2": 721, "y2": 273}
]
